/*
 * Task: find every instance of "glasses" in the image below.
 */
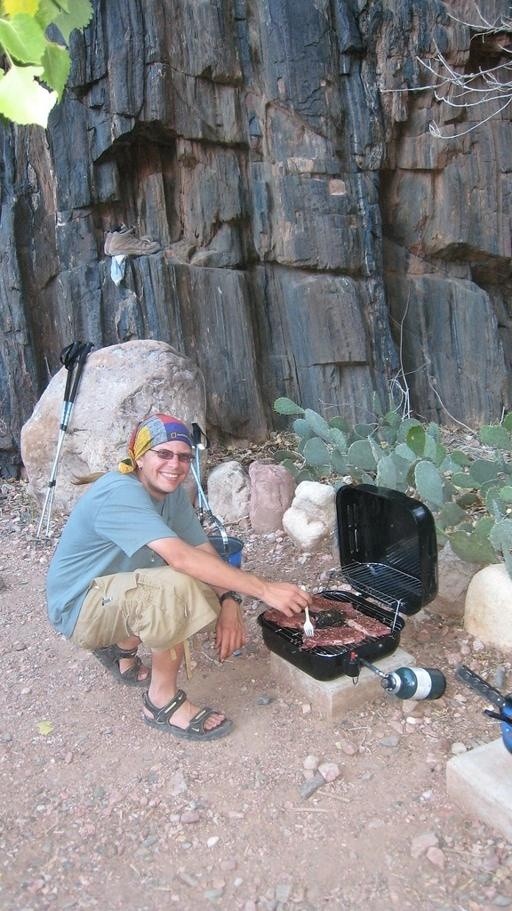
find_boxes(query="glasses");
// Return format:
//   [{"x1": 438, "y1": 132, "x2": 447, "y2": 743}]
[{"x1": 149, "y1": 448, "x2": 197, "y2": 465}]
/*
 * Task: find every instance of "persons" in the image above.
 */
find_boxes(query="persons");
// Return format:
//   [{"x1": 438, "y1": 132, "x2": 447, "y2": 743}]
[{"x1": 46, "y1": 412, "x2": 315, "y2": 743}]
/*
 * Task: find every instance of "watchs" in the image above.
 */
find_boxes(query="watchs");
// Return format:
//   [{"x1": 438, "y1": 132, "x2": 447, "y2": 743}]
[{"x1": 219, "y1": 589, "x2": 243, "y2": 607}]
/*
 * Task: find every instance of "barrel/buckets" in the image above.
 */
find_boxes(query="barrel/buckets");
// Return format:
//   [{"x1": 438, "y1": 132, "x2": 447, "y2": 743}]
[{"x1": 199, "y1": 515, "x2": 244, "y2": 568}]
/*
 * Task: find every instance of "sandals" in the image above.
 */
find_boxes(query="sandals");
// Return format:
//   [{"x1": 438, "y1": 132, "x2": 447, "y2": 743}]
[
  {"x1": 140, "y1": 689, "x2": 235, "y2": 742},
  {"x1": 89, "y1": 639, "x2": 153, "y2": 687}
]
